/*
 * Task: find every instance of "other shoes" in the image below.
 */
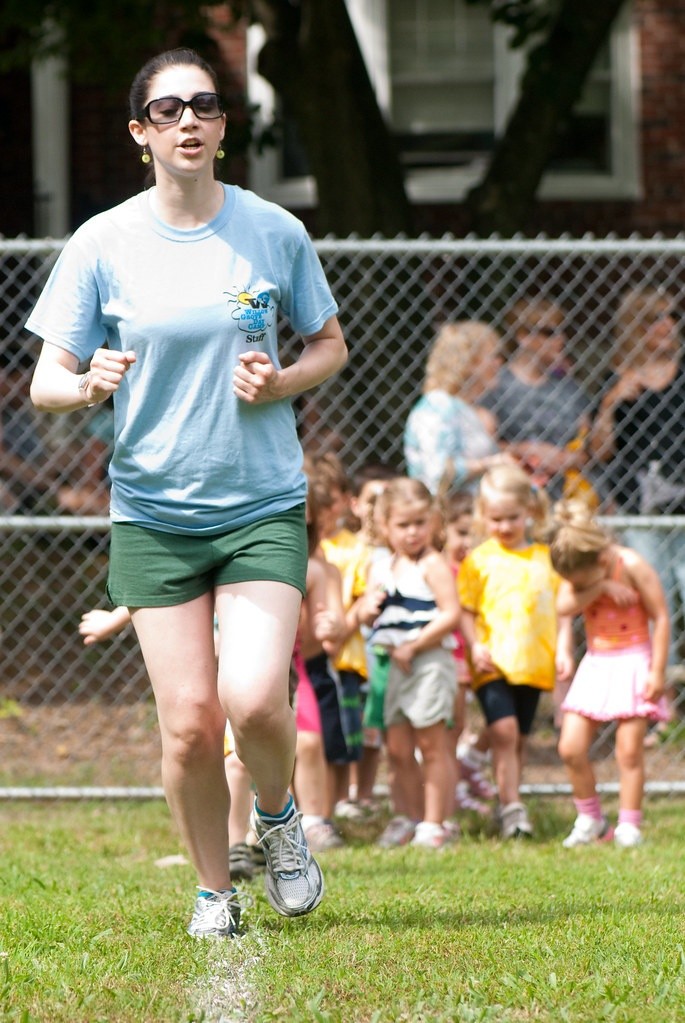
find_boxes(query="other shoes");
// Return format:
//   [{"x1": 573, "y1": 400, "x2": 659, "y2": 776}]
[{"x1": 338, "y1": 801, "x2": 382, "y2": 824}]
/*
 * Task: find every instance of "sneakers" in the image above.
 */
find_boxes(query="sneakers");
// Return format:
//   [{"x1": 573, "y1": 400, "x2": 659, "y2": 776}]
[
  {"x1": 601, "y1": 822, "x2": 641, "y2": 852},
  {"x1": 408, "y1": 825, "x2": 450, "y2": 853},
  {"x1": 453, "y1": 791, "x2": 497, "y2": 821},
  {"x1": 302, "y1": 815, "x2": 350, "y2": 849},
  {"x1": 222, "y1": 841, "x2": 256, "y2": 880},
  {"x1": 185, "y1": 886, "x2": 241, "y2": 947},
  {"x1": 251, "y1": 804, "x2": 326, "y2": 917},
  {"x1": 561, "y1": 815, "x2": 611, "y2": 850},
  {"x1": 452, "y1": 761, "x2": 495, "y2": 797},
  {"x1": 497, "y1": 806, "x2": 538, "y2": 842},
  {"x1": 370, "y1": 818, "x2": 410, "y2": 847}
]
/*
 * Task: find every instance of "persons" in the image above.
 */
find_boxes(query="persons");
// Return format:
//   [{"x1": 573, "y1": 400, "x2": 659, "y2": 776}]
[
  {"x1": 400, "y1": 318, "x2": 510, "y2": 767},
  {"x1": 22, "y1": 45, "x2": 326, "y2": 940},
  {"x1": 455, "y1": 465, "x2": 574, "y2": 842},
  {"x1": 76, "y1": 447, "x2": 499, "y2": 883},
  {"x1": 0, "y1": 337, "x2": 348, "y2": 551},
  {"x1": 587, "y1": 283, "x2": 685, "y2": 750},
  {"x1": 532, "y1": 496, "x2": 675, "y2": 852},
  {"x1": 468, "y1": 294, "x2": 617, "y2": 739}
]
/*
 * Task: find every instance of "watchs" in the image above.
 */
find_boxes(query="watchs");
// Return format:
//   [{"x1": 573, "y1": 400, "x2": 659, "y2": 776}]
[{"x1": 77, "y1": 370, "x2": 113, "y2": 408}]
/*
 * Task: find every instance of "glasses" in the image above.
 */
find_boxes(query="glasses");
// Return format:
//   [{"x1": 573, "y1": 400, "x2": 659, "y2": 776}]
[
  {"x1": 137, "y1": 93, "x2": 228, "y2": 126},
  {"x1": 524, "y1": 326, "x2": 573, "y2": 338}
]
